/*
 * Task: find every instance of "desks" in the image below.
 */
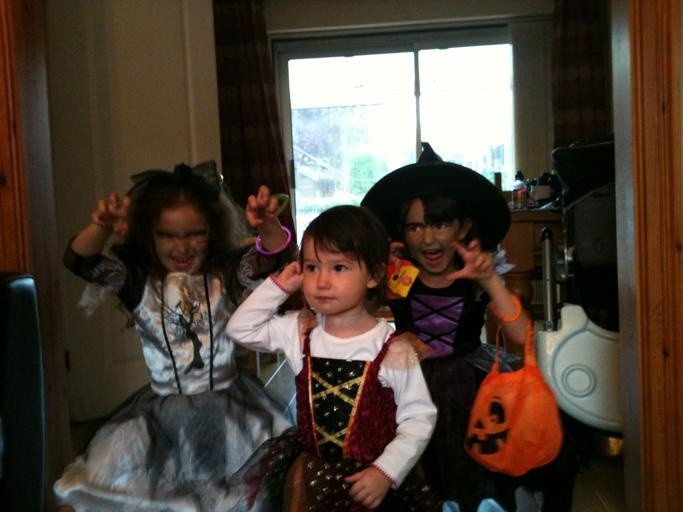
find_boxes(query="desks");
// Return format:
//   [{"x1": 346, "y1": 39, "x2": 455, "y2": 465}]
[{"x1": 502, "y1": 205, "x2": 559, "y2": 306}]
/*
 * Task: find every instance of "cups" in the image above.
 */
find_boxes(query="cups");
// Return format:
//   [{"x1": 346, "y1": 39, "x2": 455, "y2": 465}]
[{"x1": 530, "y1": 185, "x2": 550, "y2": 202}]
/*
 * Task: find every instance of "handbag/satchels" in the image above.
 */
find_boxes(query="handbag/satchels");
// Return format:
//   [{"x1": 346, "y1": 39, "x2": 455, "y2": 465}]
[{"x1": 464, "y1": 320, "x2": 562, "y2": 477}]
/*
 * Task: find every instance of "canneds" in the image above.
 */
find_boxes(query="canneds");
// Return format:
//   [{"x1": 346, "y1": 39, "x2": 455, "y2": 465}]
[{"x1": 513, "y1": 186, "x2": 526, "y2": 209}]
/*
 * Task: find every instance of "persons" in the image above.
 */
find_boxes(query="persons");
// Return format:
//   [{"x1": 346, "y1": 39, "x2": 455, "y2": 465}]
[
  {"x1": 54, "y1": 161, "x2": 298, "y2": 511},
  {"x1": 360, "y1": 143, "x2": 533, "y2": 511},
  {"x1": 225, "y1": 205, "x2": 437, "y2": 512}
]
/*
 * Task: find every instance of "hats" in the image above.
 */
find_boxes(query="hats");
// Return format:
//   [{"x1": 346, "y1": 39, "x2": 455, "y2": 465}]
[{"x1": 360, "y1": 142, "x2": 511, "y2": 251}]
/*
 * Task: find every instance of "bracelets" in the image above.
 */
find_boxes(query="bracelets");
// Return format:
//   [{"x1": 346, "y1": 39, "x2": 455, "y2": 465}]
[
  {"x1": 256, "y1": 226, "x2": 291, "y2": 254},
  {"x1": 491, "y1": 294, "x2": 522, "y2": 321}
]
